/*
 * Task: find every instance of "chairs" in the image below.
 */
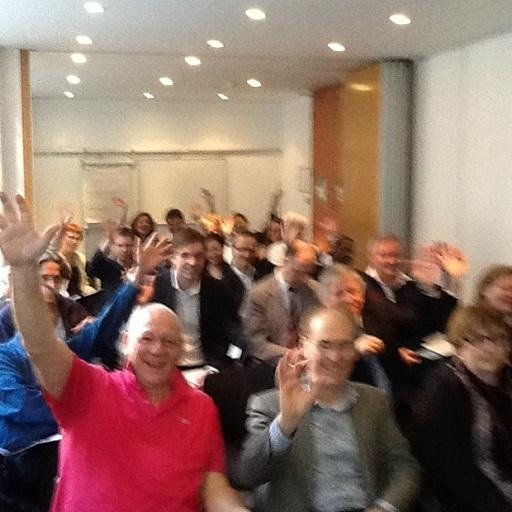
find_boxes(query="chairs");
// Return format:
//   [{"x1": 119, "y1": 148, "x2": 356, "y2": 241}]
[{"x1": 199, "y1": 366, "x2": 274, "y2": 438}]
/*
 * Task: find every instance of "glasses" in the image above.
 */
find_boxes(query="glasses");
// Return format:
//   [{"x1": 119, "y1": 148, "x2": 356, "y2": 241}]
[{"x1": 464, "y1": 333, "x2": 511, "y2": 347}]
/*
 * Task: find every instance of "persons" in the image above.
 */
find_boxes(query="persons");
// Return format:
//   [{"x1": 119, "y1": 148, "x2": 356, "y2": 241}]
[{"x1": 0, "y1": 188, "x2": 510, "y2": 512}]
